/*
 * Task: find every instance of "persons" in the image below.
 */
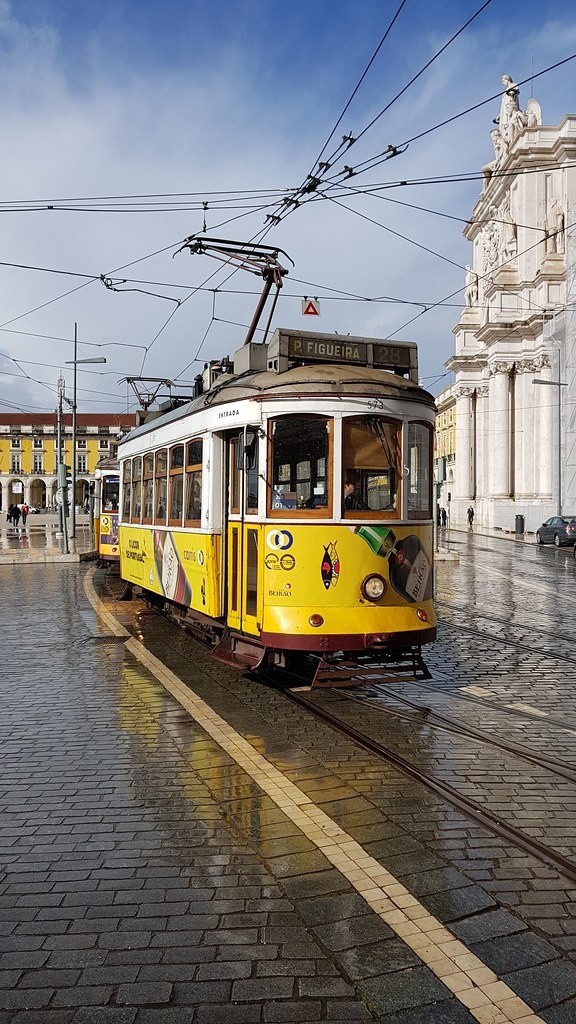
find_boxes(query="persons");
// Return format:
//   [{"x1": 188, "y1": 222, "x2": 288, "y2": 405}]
[
  {"x1": 343, "y1": 479, "x2": 370, "y2": 511},
  {"x1": 496, "y1": 74, "x2": 520, "y2": 136},
  {"x1": 6, "y1": 503, "x2": 21, "y2": 527},
  {"x1": 21, "y1": 503, "x2": 29, "y2": 527},
  {"x1": 467, "y1": 505, "x2": 474, "y2": 526},
  {"x1": 74, "y1": 492, "x2": 90, "y2": 514},
  {"x1": 441, "y1": 508, "x2": 447, "y2": 526},
  {"x1": 104, "y1": 497, "x2": 113, "y2": 510},
  {"x1": 436, "y1": 504, "x2": 441, "y2": 527}
]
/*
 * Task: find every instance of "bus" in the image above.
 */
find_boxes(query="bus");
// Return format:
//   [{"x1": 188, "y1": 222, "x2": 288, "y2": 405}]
[
  {"x1": 118, "y1": 327, "x2": 437, "y2": 688},
  {"x1": 89, "y1": 457, "x2": 120, "y2": 561}
]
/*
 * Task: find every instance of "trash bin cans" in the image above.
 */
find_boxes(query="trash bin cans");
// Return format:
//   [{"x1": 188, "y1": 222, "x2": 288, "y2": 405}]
[{"x1": 514, "y1": 514, "x2": 525, "y2": 534}]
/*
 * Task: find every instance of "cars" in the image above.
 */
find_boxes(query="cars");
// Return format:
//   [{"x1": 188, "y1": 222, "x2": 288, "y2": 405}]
[{"x1": 536, "y1": 515, "x2": 576, "y2": 547}]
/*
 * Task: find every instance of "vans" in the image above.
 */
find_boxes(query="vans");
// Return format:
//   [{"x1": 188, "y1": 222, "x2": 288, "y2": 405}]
[{"x1": 17, "y1": 504, "x2": 41, "y2": 514}]
